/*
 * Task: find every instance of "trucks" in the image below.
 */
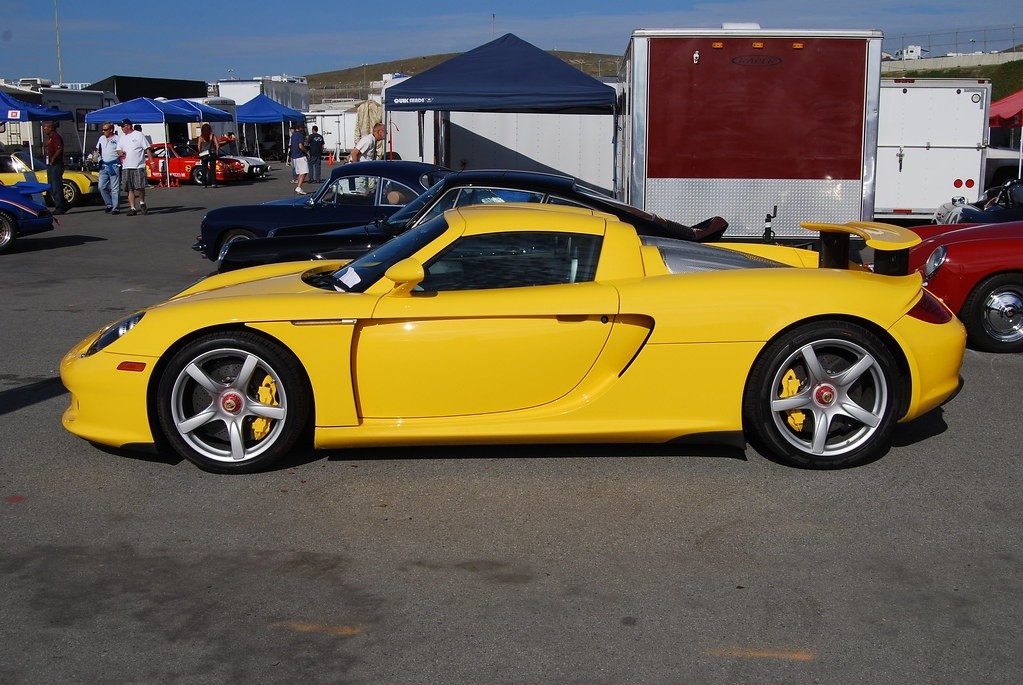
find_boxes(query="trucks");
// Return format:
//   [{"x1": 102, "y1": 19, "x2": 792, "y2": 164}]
[
  {"x1": 0, "y1": 77, "x2": 121, "y2": 157},
  {"x1": 610, "y1": 24, "x2": 992, "y2": 241}
]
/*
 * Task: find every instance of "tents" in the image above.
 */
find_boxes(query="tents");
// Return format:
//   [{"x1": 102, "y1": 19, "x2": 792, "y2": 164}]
[
  {"x1": 0, "y1": 89, "x2": 89, "y2": 171},
  {"x1": 237, "y1": 94, "x2": 306, "y2": 158},
  {"x1": 385, "y1": 33, "x2": 618, "y2": 200},
  {"x1": 82, "y1": 97, "x2": 240, "y2": 188}
]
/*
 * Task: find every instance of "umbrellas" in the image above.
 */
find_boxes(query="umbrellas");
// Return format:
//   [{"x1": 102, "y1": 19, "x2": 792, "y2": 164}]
[{"x1": 989, "y1": 91, "x2": 1023, "y2": 179}]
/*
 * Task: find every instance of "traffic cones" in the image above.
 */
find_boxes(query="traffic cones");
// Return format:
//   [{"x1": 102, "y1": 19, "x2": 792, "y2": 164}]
[{"x1": 329, "y1": 153, "x2": 334, "y2": 165}]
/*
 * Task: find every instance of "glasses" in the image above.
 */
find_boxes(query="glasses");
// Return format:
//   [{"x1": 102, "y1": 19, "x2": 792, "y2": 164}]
[{"x1": 102, "y1": 128, "x2": 110, "y2": 131}]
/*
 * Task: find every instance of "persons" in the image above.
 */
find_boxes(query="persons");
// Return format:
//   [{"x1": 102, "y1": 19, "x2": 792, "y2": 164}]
[
  {"x1": 346, "y1": 123, "x2": 386, "y2": 195},
  {"x1": 134, "y1": 125, "x2": 155, "y2": 189},
  {"x1": 116, "y1": 118, "x2": 156, "y2": 215},
  {"x1": 198, "y1": 124, "x2": 219, "y2": 188},
  {"x1": 43, "y1": 122, "x2": 72, "y2": 215},
  {"x1": 290, "y1": 123, "x2": 324, "y2": 195},
  {"x1": 96, "y1": 122, "x2": 122, "y2": 215}
]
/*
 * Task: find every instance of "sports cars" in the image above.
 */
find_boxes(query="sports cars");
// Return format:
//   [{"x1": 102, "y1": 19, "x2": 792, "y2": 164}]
[{"x1": 57, "y1": 201, "x2": 968, "y2": 475}]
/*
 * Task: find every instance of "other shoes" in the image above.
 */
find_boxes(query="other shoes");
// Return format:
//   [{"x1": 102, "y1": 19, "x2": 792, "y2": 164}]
[
  {"x1": 139, "y1": 203, "x2": 148, "y2": 215},
  {"x1": 52, "y1": 205, "x2": 67, "y2": 215},
  {"x1": 308, "y1": 179, "x2": 321, "y2": 184},
  {"x1": 289, "y1": 180, "x2": 298, "y2": 184},
  {"x1": 127, "y1": 209, "x2": 137, "y2": 216},
  {"x1": 105, "y1": 207, "x2": 113, "y2": 213},
  {"x1": 202, "y1": 184, "x2": 217, "y2": 188},
  {"x1": 65, "y1": 204, "x2": 72, "y2": 212},
  {"x1": 111, "y1": 211, "x2": 120, "y2": 215},
  {"x1": 294, "y1": 186, "x2": 307, "y2": 195}
]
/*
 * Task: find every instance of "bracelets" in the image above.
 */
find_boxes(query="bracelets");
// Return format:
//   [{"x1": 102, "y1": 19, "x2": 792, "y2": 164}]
[{"x1": 150, "y1": 162, "x2": 155, "y2": 164}]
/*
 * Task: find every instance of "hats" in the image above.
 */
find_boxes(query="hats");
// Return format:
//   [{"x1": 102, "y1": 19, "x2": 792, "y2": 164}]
[
  {"x1": 118, "y1": 118, "x2": 132, "y2": 126},
  {"x1": 289, "y1": 121, "x2": 307, "y2": 129}
]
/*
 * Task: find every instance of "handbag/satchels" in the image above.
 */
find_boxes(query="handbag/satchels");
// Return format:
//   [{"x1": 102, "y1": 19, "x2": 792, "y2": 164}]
[{"x1": 198, "y1": 150, "x2": 212, "y2": 161}]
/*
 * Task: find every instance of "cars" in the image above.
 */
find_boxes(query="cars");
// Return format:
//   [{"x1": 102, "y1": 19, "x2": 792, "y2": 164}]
[
  {"x1": 145, "y1": 144, "x2": 272, "y2": 186},
  {"x1": 192, "y1": 160, "x2": 729, "y2": 273},
  {"x1": 0, "y1": 144, "x2": 100, "y2": 252},
  {"x1": 908, "y1": 179, "x2": 1023, "y2": 353}
]
